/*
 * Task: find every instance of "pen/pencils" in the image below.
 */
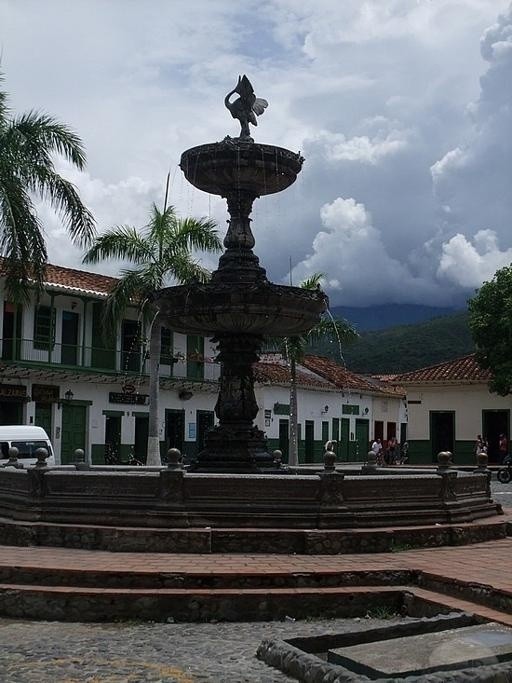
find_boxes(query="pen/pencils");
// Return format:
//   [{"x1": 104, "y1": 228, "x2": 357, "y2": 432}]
[{"x1": 0, "y1": 424, "x2": 56, "y2": 470}]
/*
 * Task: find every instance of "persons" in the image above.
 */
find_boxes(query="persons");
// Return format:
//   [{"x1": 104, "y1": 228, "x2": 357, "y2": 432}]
[
  {"x1": 371, "y1": 431, "x2": 409, "y2": 467},
  {"x1": 482, "y1": 436, "x2": 489, "y2": 453},
  {"x1": 497, "y1": 433, "x2": 507, "y2": 464},
  {"x1": 472, "y1": 434, "x2": 484, "y2": 455},
  {"x1": 323, "y1": 439, "x2": 337, "y2": 453}
]
